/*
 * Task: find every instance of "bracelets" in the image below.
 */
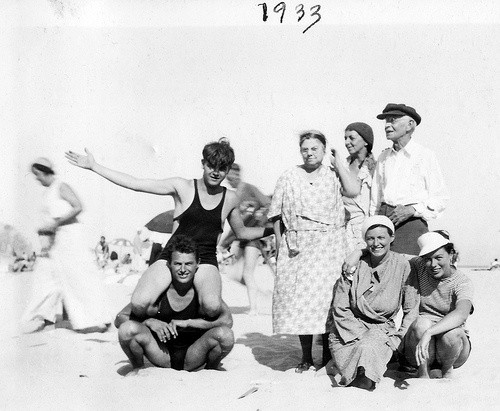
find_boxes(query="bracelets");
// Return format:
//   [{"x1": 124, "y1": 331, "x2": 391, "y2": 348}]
[{"x1": 391, "y1": 334, "x2": 403, "y2": 342}]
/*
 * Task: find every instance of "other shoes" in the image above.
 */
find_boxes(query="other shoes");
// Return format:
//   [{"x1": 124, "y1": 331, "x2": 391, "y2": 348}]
[{"x1": 295, "y1": 361, "x2": 311, "y2": 373}]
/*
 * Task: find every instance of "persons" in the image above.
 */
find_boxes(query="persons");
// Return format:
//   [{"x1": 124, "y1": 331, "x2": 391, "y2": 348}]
[
  {"x1": 218, "y1": 164, "x2": 277, "y2": 317},
  {"x1": 266, "y1": 129, "x2": 360, "y2": 372},
  {"x1": 8, "y1": 251, "x2": 37, "y2": 273},
  {"x1": 368, "y1": 103, "x2": 447, "y2": 257},
  {"x1": 14, "y1": 159, "x2": 131, "y2": 344},
  {"x1": 340, "y1": 122, "x2": 377, "y2": 237},
  {"x1": 328, "y1": 214, "x2": 420, "y2": 390},
  {"x1": 95, "y1": 230, "x2": 156, "y2": 285},
  {"x1": 114, "y1": 235, "x2": 234, "y2": 378},
  {"x1": 342, "y1": 231, "x2": 476, "y2": 379},
  {"x1": 64, "y1": 137, "x2": 287, "y2": 320}
]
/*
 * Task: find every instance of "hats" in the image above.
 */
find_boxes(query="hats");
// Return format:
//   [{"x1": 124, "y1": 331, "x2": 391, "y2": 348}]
[
  {"x1": 346, "y1": 122, "x2": 374, "y2": 153},
  {"x1": 361, "y1": 214, "x2": 395, "y2": 242},
  {"x1": 417, "y1": 232, "x2": 452, "y2": 257},
  {"x1": 32, "y1": 156, "x2": 56, "y2": 174},
  {"x1": 376, "y1": 103, "x2": 422, "y2": 126}
]
